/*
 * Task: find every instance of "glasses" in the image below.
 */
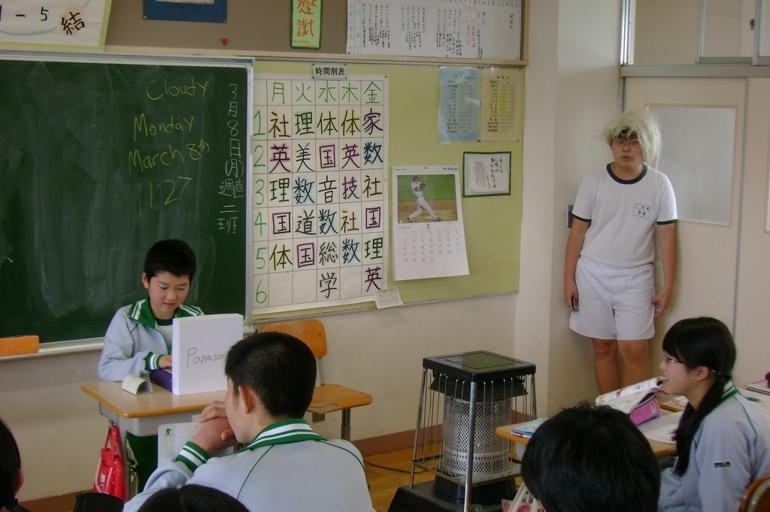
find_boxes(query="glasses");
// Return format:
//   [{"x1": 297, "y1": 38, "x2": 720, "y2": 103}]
[{"x1": 664, "y1": 356, "x2": 681, "y2": 365}]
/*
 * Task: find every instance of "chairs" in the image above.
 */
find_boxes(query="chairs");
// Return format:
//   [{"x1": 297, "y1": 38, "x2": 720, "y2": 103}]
[
  {"x1": 255, "y1": 319, "x2": 374, "y2": 441},
  {"x1": 736, "y1": 471, "x2": 770, "y2": 512}
]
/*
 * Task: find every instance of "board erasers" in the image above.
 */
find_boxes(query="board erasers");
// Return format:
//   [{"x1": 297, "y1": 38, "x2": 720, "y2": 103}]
[{"x1": 0, "y1": 335, "x2": 39, "y2": 357}]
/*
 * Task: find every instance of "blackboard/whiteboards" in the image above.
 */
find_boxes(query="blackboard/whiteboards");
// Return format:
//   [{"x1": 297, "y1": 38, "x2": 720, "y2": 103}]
[{"x1": 0, "y1": 50, "x2": 254, "y2": 361}]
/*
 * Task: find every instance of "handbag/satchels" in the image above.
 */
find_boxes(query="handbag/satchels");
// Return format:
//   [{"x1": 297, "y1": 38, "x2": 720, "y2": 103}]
[{"x1": 95, "y1": 426, "x2": 128, "y2": 500}]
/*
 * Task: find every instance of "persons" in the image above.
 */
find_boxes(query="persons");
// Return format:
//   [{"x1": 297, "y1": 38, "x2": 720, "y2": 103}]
[
  {"x1": 564, "y1": 108, "x2": 678, "y2": 395},
  {"x1": 1, "y1": 422, "x2": 29, "y2": 512},
  {"x1": 98, "y1": 239, "x2": 207, "y2": 492},
  {"x1": 520, "y1": 403, "x2": 660, "y2": 510},
  {"x1": 654, "y1": 318, "x2": 769, "y2": 512},
  {"x1": 123, "y1": 331, "x2": 376, "y2": 512},
  {"x1": 136, "y1": 484, "x2": 248, "y2": 512},
  {"x1": 406, "y1": 175, "x2": 443, "y2": 222}
]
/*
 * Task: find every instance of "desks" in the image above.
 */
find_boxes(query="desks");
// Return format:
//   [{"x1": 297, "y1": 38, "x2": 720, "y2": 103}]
[
  {"x1": 494, "y1": 409, "x2": 679, "y2": 499},
  {"x1": 745, "y1": 380, "x2": 770, "y2": 396},
  {"x1": 79, "y1": 380, "x2": 237, "y2": 504},
  {"x1": 658, "y1": 386, "x2": 770, "y2": 414}
]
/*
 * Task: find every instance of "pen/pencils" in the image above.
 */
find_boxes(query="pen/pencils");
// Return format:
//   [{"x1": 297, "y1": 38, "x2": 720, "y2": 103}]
[{"x1": 744, "y1": 396, "x2": 760, "y2": 401}]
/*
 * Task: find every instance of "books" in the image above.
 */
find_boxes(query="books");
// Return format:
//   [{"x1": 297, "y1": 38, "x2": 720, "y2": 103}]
[
  {"x1": 744, "y1": 381, "x2": 768, "y2": 395},
  {"x1": 513, "y1": 418, "x2": 547, "y2": 439},
  {"x1": 595, "y1": 377, "x2": 664, "y2": 415}
]
